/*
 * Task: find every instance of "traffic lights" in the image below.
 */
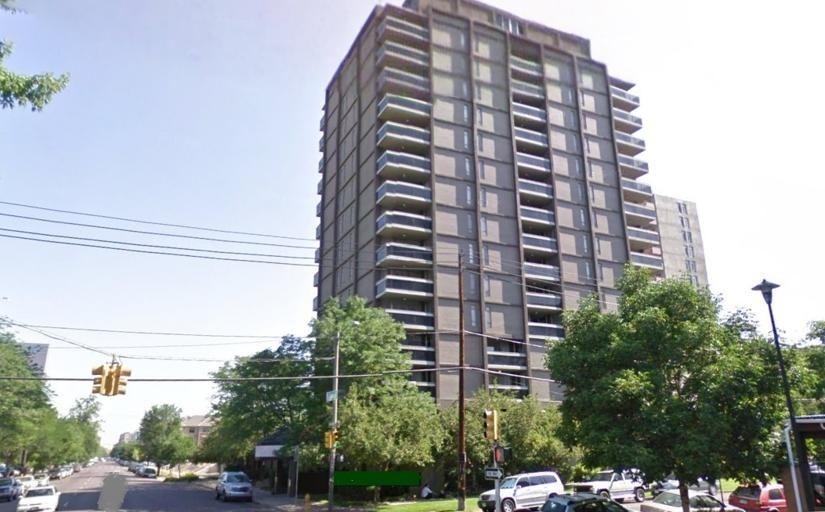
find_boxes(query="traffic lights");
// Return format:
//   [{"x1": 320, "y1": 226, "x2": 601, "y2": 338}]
[
  {"x1": 494, "y1": 446, "x2": 513, "y2": 465},
  {"x1": 90, "y1": 359, "x2": 132, "y2": 397},
  {"x1": 482, "y1": 408, "x2": 500, "y2": 441},
  {"x1": 324, "y1": 428, "x2": 339, "y2": 449}
]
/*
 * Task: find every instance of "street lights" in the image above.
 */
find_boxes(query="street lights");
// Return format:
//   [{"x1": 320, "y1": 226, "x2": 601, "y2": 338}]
[
  {"x1": 751, "y1": 280, "x2": 819, "y2": 511},
  {"x1": 324, "y1": 315, "x2": 361, "y2": 512}
]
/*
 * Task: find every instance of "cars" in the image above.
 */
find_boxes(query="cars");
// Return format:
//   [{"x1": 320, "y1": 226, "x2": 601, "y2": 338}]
[
  {"x1": 214, "y1": 471, "x2": 253, "y2": 502},
  {"x1": 111, "y1": 457, "x2": 159, "y2": 477},
  {"x1": 0, "y1": 454, "x2": 106, "y2": 512}
]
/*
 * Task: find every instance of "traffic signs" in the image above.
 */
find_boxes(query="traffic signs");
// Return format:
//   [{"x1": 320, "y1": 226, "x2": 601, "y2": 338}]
[{"x1": 482, "y1": 467, "x2": 506, "y2": 482}]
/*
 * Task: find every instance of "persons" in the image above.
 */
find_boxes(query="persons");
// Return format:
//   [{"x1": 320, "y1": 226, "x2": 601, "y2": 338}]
[{"x1": 420, "y1": 482, "x2": 433, "y2": 499}]
